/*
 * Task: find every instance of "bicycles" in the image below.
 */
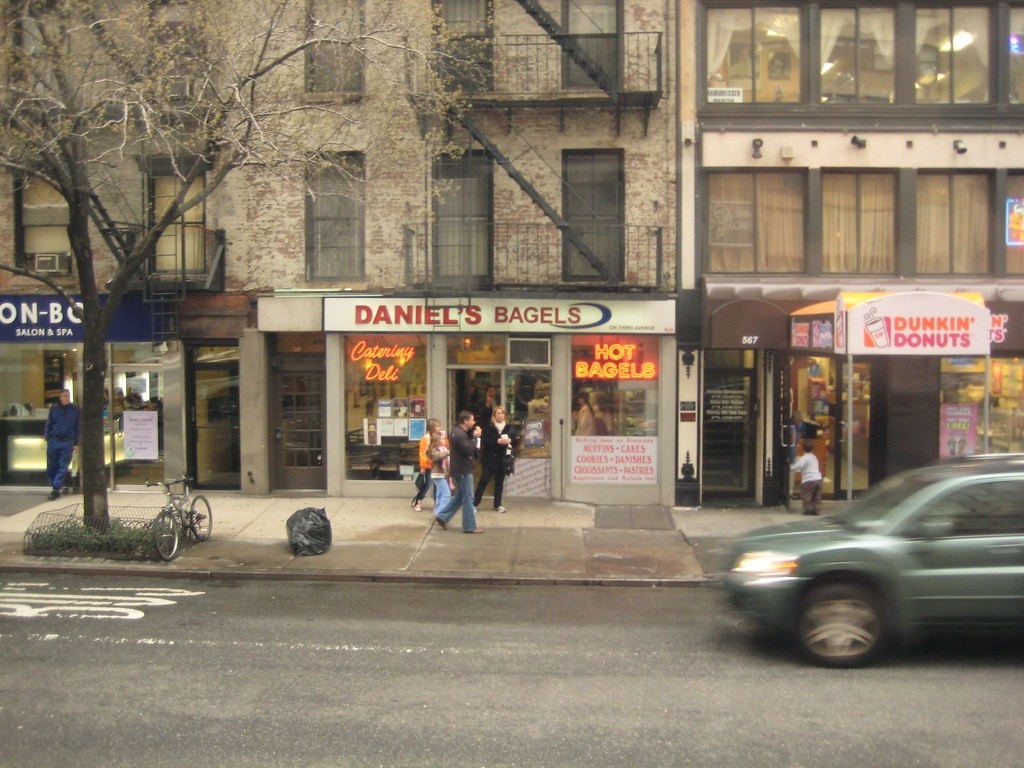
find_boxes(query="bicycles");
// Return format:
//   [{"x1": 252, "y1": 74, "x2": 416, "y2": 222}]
[{"x1": 145, "y1": 472, "x2": 213, "y2": 561}]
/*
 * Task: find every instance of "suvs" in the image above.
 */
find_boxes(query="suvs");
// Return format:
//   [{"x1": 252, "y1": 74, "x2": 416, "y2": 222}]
[{"x1": 719, "y1": 450, "x2": 1024, "y2": 668}]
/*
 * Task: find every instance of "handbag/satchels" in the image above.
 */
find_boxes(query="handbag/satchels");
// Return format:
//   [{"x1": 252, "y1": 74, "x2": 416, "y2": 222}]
[
  {"x1": 595, "y1": 417, "x2": 609, "y2": 436},
  {"x1": 415, "y1": 472, "x2": 426, "y2": 490},
  {"x1": 502, "y1": 451, "x2": 514, "y2": 476}
]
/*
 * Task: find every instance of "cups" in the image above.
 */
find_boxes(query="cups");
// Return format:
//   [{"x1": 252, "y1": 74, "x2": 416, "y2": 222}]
[
  {"x1": 865, "y1": 317, "x2": 890, "y2": 347},
  {"x1": 501, "y1": 434, "x2": 508, "y2": 439}
]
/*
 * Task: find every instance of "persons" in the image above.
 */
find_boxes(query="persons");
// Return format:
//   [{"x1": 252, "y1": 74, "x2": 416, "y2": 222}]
[
  {"x1": 473, "y1": 407, "x2": 516, "y2": 513},
  {"x1": 514, "y1": 371, "x2": 537, "y2": 418},
  {"x1": 44, "y1": 390, "x2": 80, "y2": 500},
  {"x1": 789, "y1": 439, "x2": 823, "y2": 515},
  {"x1": 413, "y1": 419, "x2": 449, "y2": 511},
  {"x1": 462, "y1": 385, "x2": 497, "y2": 458},
  {"x1": 434, "y1": 411, "x2": 484, "y2": 534},
  {"x1": 427, "y1": 433, "x2": 452, "y2": 514},
  {"x1": 114, "y1": 387, "x2": 163, "y2": 424},
  {"x1": 573, "y1": 385, "x2": 612, "y2": 437}
]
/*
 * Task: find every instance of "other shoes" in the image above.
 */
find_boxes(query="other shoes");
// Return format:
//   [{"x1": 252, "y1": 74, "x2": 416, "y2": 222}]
[
  {"x1": 474, "y1": 506, "x2": 477, "y2": 513},
  {"x1": 48, "y1": 488, "x2": 59, "y2": 499},
  {"x1": 414, "y1": 499, "x2": 422, "y2": 512},
  {"x1": 469, "y1": 529, "x2": 484, "y2": 533},
  {"x1": 435, "y1": 516, "x2": 447, "y2": 530},
  {"x1": 494, "y1": 506, "x2": 506, "y2": 512}
]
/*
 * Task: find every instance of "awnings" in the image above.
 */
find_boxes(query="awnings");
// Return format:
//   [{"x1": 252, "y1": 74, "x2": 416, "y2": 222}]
[{"x1": 701, "y1": 276, "x2": 1024, "y2": 352}]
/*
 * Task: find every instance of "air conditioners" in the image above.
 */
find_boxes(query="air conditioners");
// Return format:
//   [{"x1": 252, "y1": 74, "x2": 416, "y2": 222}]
[{"x1": 35, "y1": 254, "x2": 69, "y2": 276}]
